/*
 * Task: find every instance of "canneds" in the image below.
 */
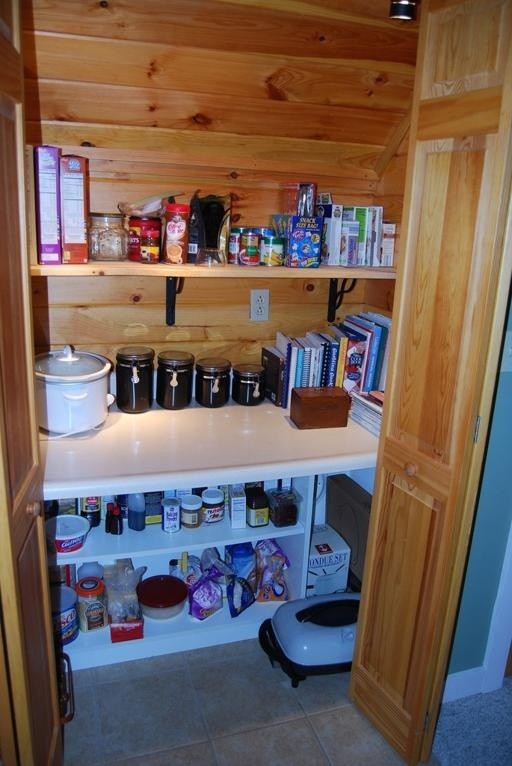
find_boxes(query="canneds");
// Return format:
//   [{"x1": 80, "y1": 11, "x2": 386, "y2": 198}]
[
  {"x1": 161, "y1": 488, "x2": 225, "y2": 532},
  {"x1": 87, "y1": 212, "x2": 286, "y2": 267},
  {"x1": 51, "y1": 574, "x2": 107, "y2": 647}
]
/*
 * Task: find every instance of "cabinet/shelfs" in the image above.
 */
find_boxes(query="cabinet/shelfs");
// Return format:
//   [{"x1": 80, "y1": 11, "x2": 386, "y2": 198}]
[{"x1": 29, "y1": 257, "x2": 400, "y2": 668}]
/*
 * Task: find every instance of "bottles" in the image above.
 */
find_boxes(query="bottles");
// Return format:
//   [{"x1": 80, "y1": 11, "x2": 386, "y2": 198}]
[
  {"x1": 140, "y1": 231, "x2": 159, "y2": 265},
  {"x1": 105, "y1": 503, "x2": 122, "y2": 535},
  {"x1": 161, "y1": 204, "x2": 192, "y2": 267},
  {"x1": 74, "y1": 498, "x2": 101, "y2": 528},
  {"x1": 178, "y1": 493, "x2": 202, "y2": 529},
  {"x1": 88, "y1": 210, "x2": 129, "y2": 262},
  {"x1": 201, "y1": 488, "x2": 226, "y2": 524}
]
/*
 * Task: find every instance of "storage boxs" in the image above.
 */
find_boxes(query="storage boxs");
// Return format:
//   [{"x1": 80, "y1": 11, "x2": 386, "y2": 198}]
[{"x1": 306, "y1": 522, "x2": 351, "y2": 597}]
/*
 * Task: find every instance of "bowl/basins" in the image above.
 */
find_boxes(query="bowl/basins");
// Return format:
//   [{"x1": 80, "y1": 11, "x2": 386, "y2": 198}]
[
  {"x1": 46, "y1": 514, "x2": 90, "y2": 555},
  {"x1": 136, "y1": 573, "x2": 188, "y2": 621}
]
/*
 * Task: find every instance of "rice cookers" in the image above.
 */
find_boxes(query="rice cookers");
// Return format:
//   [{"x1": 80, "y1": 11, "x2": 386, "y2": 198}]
[{"x1": 33, "y1": 343, "x2": 117, "y2": 440}]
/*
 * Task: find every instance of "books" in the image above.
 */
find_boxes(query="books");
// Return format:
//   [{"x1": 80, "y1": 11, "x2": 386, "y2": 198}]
[{"x1": 259, "y1": 311, "x2": 394, "y2": 445}]
